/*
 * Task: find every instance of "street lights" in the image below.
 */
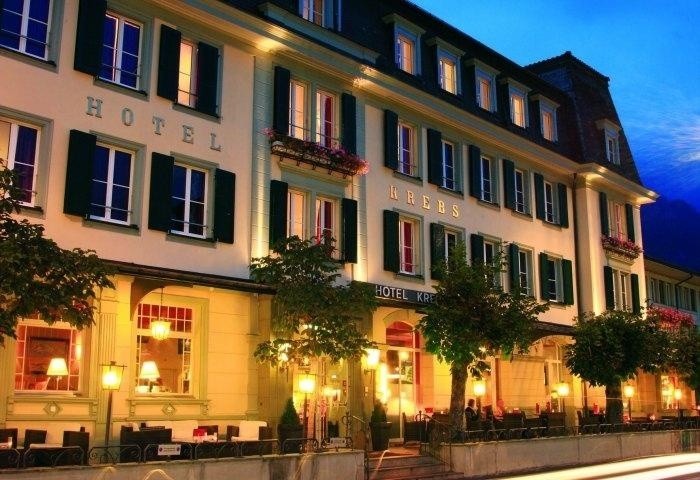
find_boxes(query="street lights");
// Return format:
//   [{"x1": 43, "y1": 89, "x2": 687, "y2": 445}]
[
  {"x1": 624, "y1": 385, "x2": 635, "y2": 423},
  {"x1": 300, "y1": 380, "x2": 313, "y2": 450},
  {"x1": 674, "y1": 389, "x2": 682, "y2": 421},
  {"x1": 474, "y1": 382, "x2": 486, "y2": 414},
  {"x1": 99, "y1": 359, "x2": 127, "y2": 463},
  {"x1": 557, "y1": 382, "x2": 569, "y2": 431}
]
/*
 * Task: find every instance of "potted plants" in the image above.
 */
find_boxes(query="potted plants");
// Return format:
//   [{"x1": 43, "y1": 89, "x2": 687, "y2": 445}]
[
  {"x1": 369, "y1": 399, "x2": 391, "y2": 450},
  {"x1": 277, "y1": 397, "x2": 304, "y2": 452}
]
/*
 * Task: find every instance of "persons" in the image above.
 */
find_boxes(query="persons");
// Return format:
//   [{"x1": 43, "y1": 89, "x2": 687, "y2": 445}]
[
  {"x1": 465, "y1": 399, "x2": 481, "y2": 425},
  {"x1": 492, "y1": 399, "x2": 512, "y2": 417}
]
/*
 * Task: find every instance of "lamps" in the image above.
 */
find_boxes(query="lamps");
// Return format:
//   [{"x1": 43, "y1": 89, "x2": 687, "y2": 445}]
[
  {"x1": 151, "y1": 289, "x2": 171, "y2": 342},
  {"x1": 47, "y1": 357, "x2": 69, "y2": 389},
  {"x1": 139, "y1": 361, "x2": 161, "y2": 393}
]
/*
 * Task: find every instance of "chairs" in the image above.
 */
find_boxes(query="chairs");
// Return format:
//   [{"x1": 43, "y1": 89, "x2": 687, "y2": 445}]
[
  {"x1": 467, "y1": 412, "x2": 700, "y2": 440},
  {"x1": 1, "y1": 427, "x2": 91, "y2": 467},
  {"x1": 119, "y1": 419, "x2": 272, "y2": 463}
]
